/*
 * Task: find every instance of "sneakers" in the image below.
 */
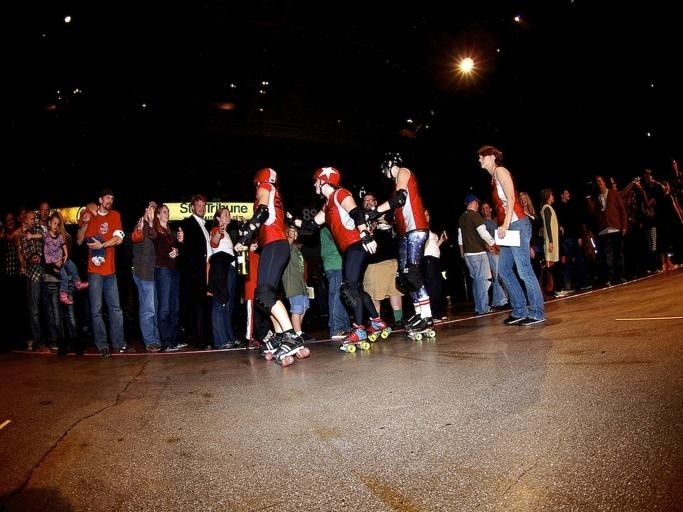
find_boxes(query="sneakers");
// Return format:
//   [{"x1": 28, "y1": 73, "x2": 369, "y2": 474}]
[
  {"x1": 330, "y1": 330, "x2": 350, "y2": 339},
  {"x1": 503, "y1": 315, "x2": 524, "y2": 324},
  {"x1": 521, "y1": 317, "x2": 545, "y2": 325},
  {"x1": 147, "y1": 343, "x2": 160, "y2": 352},
  {"x1": 59, "y1": 290, "x2": 73, "y2": 305},
  {"x1": 99, "y1": 349, "x2": 111, "y2": 357},
  {"x1": 91, "y1": 257, "x2": 101, "y2": 267},
  {"x1": 219, "y1": 342, "x2": 233, "y2": 349},
  {"x1": 299, "y1": 333, "x2": 315, "y2": 342},
  {"x1": 272, "y1": 333, "x2": 310, "y2": 366},
  {"x1": 164, "y1": 345, "x2": 179, "y2": 352},
  {"x1": 232, "y1": 338, "x2": 241, "y2": 345},
  {"x1": 176, "y1": 342, "x2": 188, "y2": 348},
  {"x1": 259, "y1": 334, "x2": 283, "y2": 360},
  {"x1": 98, "y1": 256, "x2": 105, "y2": 263},
  {"x1": 116, "y1": 345, "x2": 136, "y2": 353}
]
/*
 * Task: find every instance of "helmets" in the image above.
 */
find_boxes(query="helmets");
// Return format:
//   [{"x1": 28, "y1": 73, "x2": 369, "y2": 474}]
[
  {"x1": 313, "y1": 166, "x2": 341, "y2": 187},
  {"x1": 253, "y1": 167, "x2": 278, "y2": 184},
  {"x1": 379, "y1": 151, "x2": 402, "y2": 165}
]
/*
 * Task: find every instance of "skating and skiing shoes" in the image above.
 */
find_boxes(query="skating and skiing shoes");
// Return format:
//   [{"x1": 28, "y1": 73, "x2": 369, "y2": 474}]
[
  {"x1": 408, "y1": 317, "x2": 435, "y2": 341},
  {"x1": 405, "y1": 314, "x2": 421, "y2": 336},
  {"x1": 339, "y1": 324, "x2": 370, "y2": 352},
  {"x1": 75, "y1": 281, "x2": 89, "y2": 289},
  {"x1": 366, "y1": 317, "x2": 391, "y2": 342}
]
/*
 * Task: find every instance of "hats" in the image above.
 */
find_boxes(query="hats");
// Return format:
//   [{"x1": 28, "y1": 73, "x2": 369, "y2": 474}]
[{"x1": 465, "y1": 195, "x2": 480, "y2": 202}]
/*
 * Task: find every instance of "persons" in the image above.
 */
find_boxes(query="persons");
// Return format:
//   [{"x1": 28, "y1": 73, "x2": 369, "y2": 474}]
[
  {"x1": 282, "y1": 222, "x2": 316, "y2": 344},
  {"x1": 295, "y1": 227, "x2": 309, "y2": 283},
  {"x1": 604, "y1": 178, "x2": 634, "y2": 206},
  {"x1": 49, "y1": 212, "x2": 87, "y2": 351},
  {"x1": 481, "y1": 202, "x2": 512, "y2": 309},
  {"x1": 588, "y1": 176, "x2": 628, "y2": 286},
  {"x1": 634, "y1": 170, "x2": 683, "y2": 273},
  {"x1": 17, "y1": 211, "x2": 61, "y2": 278},
  {"x1": 367, "y1": 151, "x2": 437, "y2": 341},
  {"x1": 320, "y1": 225, "x2": 354, "y2": 340},
  {"x1": 458, "y1": 195, "x2": 499, "y2": 314},
  {"x1": 245, "y1": 233, "x2": 262, "y2": 351},
  {"x1": 27, "y1": 202, "x2": 60, "y2": 350},
  {"x1": 478, "y1": 146, "x2": 547, "y2": 326},
  {"x1": 177, "y1": 194, "x2": 213, "y2": 348},
  {"x1": 209, "y1": 208, "x2": 241, "y2": 349},
  {"x1": 558, "y1": 190, "x2": 593, "y2": 291},
  {"x1": 17, "y1": 209, "x2": 26, "y2": 223},
  {"x1": 131, "y1": 201, "x2": 162, "y2": 352},
  {"x1": 148, "y1": 206, "x2": 188, "y2": 352},
  {"x1": 670, "y1": 160, "x2": 683, "y2": 197},
  {"x1": 0, "y1": 211, "x2": 19, "y2": 276},
  {"x1": 312, "y1": 166, "x2": 392, "y2": 354},
  {"x1": 238, "y1": 168, "x2": 311, "y2": 367},
  {"x1": 422, "y1": 207, "x2": 448, "y2": 323},
  {"x1": 540, "y1": 188, "x2": 569, "y2": 297},
  {"x1": 518, "y1": 191, "x2": 536, "y2": 219},
  {"x1": 25, "y1": 213, "x2": 90, "y2": 305},
  {"x1": 79, "y1": 203, "x2": 106, "y2": 267},
  {"x1": 76, "y1": 190, "x2": 128, "y2": 359},
  {"x1": 359, "y1": 193, "x2": 406, "y2": 329}
]
